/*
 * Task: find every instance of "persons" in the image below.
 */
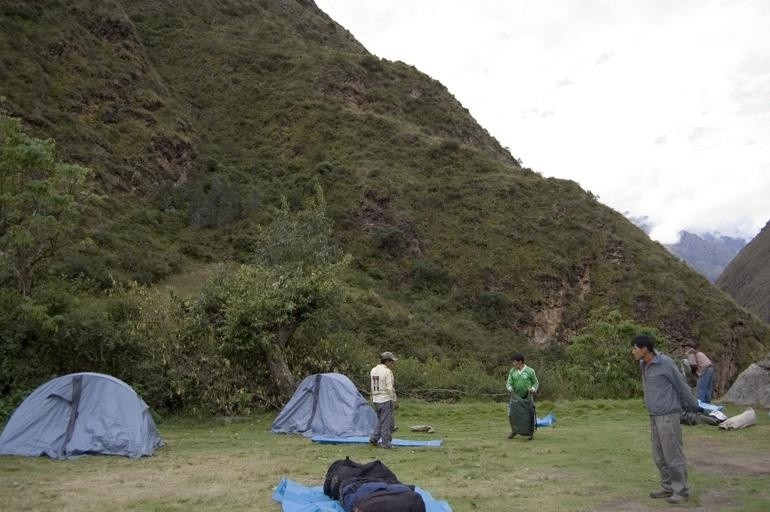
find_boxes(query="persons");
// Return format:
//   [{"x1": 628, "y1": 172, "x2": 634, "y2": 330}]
[
  {"x1": 628, "y1": 336, "x2": 701, "y2": 505},
  {"x1": 681, "y1": 339, "x2": 716, "y2": 406},
  {"x1": 366, "y1": 350, "x2": 400, "y2": 451},
  {"x1": 504, "y1": 353, "x2": 541, "y2": 442}
]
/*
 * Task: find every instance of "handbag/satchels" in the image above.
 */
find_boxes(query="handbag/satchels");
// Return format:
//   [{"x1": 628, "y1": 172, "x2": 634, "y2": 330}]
[
  {"x1": 323, "y1": 456, "x2": 400, "y2": 499},
  {"x1": 341, "y1": 479, "x2": 427, "y2": 511}
]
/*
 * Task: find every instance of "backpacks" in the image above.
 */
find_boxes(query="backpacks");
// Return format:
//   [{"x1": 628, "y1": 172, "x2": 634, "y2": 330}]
[{"x1": 509, "y1": 389, "x2": 536, "y2": 435}]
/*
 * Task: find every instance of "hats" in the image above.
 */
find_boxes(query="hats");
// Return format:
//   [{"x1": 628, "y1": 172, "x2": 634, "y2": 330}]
[{"x1": 381, "y1": 351, "x2": 399, "y2": 362}]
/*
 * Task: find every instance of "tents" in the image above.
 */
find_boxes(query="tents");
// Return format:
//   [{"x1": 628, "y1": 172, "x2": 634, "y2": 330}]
[
  {"x1": 270, "y1": 371, "x2": 382, "y2": 440},
  {"x1": 0, "y1": 370, "x2": 167, "y2": 460}
]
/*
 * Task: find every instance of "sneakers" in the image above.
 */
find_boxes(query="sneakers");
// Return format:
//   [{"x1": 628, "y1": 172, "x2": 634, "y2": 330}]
[
  {"x1": 666, "y1": 493, "x2": 690, "y2": 504},
  {"x1": 369, "y1": 438, "x2": 398, "y2": 449},
  {"x1": 650, "y1": 488, "x2": 672, "y2": 498},
  {"x1": 508, "y1": 432, "x2": 534, "y2": 440}
]
